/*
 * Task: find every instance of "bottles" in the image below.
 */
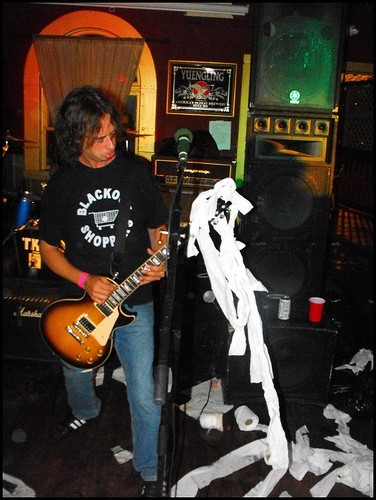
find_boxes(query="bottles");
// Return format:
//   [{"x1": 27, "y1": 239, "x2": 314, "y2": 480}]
[{"x1": 16, "y1": 191, "x2": 32, "y2": 227}]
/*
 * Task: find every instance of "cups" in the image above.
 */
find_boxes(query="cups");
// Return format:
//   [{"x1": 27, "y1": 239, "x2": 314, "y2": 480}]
[{"x1": 309, "y1": 297, "x2": 326, "y2": 322}]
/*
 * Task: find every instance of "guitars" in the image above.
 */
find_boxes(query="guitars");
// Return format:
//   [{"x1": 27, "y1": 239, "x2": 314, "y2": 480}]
[{"x1": 40, "y1": 227, "x2": 185, "y2": 372}]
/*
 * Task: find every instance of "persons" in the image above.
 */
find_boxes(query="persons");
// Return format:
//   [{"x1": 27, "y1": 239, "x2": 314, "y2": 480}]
[{"x1": 36, "y1": 85, "x2": 170, "y2": 499}]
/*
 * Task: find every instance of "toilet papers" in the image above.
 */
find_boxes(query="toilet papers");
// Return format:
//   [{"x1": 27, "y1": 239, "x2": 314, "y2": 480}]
[{"x1": 236, "y1": 405, "x2": 264, "y2": 430}]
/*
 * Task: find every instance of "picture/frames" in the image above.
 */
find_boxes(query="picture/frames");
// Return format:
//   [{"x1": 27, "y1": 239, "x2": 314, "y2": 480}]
[{"x1": 164, "y1": 58, "x2": 239, "y2": 119}]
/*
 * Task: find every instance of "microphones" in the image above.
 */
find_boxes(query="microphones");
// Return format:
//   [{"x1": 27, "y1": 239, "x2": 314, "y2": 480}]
[{"x1": 174, "y1": 128, "x2": 193, "y2": 160}]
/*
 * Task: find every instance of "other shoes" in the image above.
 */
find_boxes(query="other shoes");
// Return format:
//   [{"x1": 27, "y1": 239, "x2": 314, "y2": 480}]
[
  {"x1": 70, "y1": 400, "x2": 113, "y2": 457},
  {"x1": 137, "y1": 481, "x2": 162, "y2": 498}
]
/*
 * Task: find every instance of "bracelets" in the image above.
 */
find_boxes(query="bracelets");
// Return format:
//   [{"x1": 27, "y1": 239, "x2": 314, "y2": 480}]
[{"x1": 77, "y1": 272, "x2": 89, "y2": 288}]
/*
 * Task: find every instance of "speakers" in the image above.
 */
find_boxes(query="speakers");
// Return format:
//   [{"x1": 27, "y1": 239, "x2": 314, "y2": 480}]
[
  {"x1": 240, "y1": 155, "x2": 333, "y2": 318},
  {"x1": 224, "y1": 314, "x2": 340, "y2": 405},
  {"x1": 0, "y1": 283, "x2": 64, "y2": 363}
]
